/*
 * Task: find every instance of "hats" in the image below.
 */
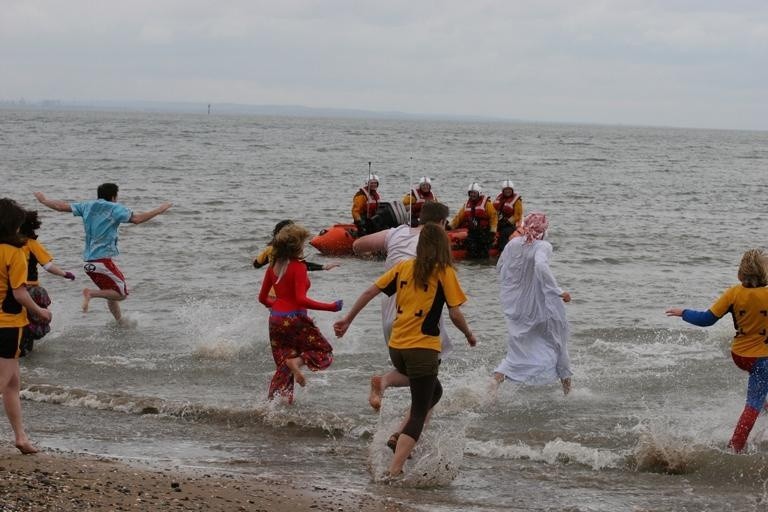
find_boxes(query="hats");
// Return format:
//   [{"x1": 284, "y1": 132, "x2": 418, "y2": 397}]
[{"x1": 24, "y1": 285, "x2": 51, "y2": 339}]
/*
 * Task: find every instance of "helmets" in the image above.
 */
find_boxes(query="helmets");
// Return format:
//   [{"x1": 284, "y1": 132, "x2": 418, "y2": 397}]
[{"x1": 366, "y1": 174, "x2": 514, "y2": 197}]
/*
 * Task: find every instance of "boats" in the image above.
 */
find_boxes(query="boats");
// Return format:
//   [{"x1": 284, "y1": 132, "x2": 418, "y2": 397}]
[{"x1": 309, "y1": 222, "x2": 524, "y2": 259}]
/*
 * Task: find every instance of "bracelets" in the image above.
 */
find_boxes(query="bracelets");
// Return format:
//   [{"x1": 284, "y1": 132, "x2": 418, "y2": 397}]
[{"x1": 465, "y1": 332, "x2": 472, "y2": 337}]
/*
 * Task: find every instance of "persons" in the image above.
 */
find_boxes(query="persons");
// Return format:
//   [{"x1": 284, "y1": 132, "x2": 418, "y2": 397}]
[
  {"x1": 665, "y1": 248, "x2": 767, "y2": 455},
  {"x1": 493, "y1": 180, "x2": 525, "y2": 256},
  {"x1": 16, "y1": 210, "x2": 75, "y2": 355},
  {"x1": 448, "y1": 183, "x2": 499, "y2": 263},
  {"x1": 487, "y1": 211, "x2": 575, "y2": 405},
  {"x1": 351, "y1": 202, "x2": 457, "y2": 410},
  {"x1": 0, "y1": 197, "x2": 53, "y2": 455},
  {"x1": 401, "y1": 177, "x2": 449, "y2": 233},
  {"x1": 352, "y1": 174, "x2": 384, "y2": 236},
  {"x1": 333, "y1": 223, "x2": 477, "y2": 479},
  {"x1": 254, "y1": 220, "x2": 341, "y2": 315},
  {"x1": 33, "y1": 183, "x2": 172, "y2": 326},
  {"x1": 259, "y1": 224, "x2": 343, "y2": 408}
]
